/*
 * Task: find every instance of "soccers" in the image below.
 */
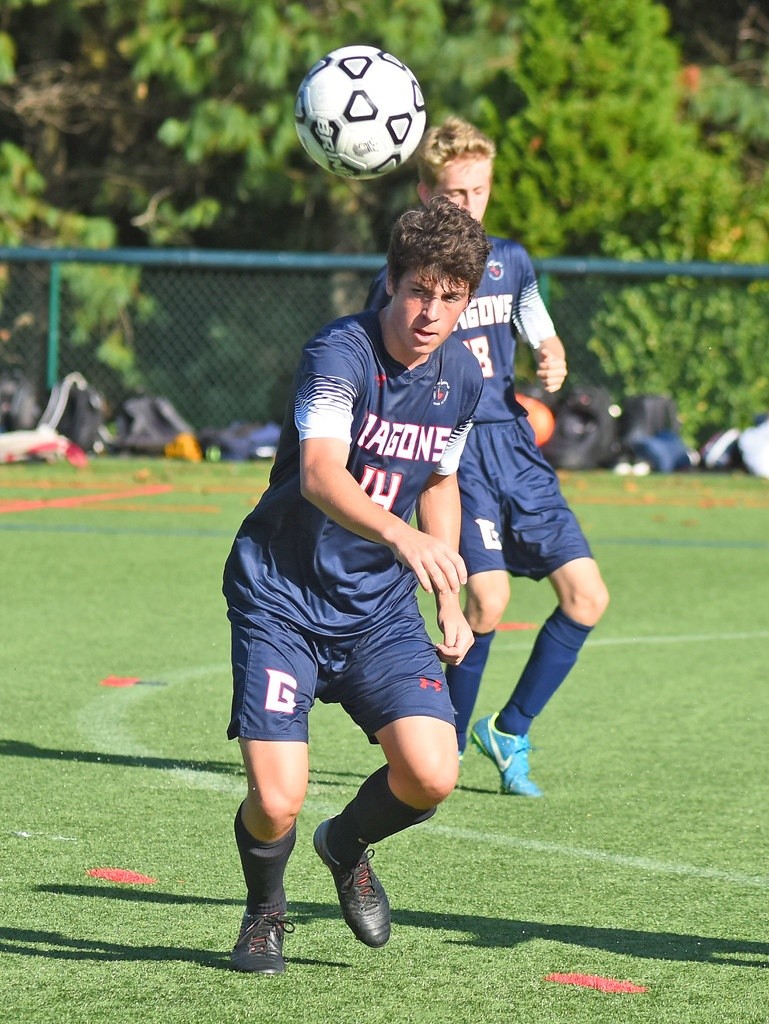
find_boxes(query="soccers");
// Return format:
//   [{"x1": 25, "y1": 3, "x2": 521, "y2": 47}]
[{"x1": 294, "y1": 45, "x2": 426, "y2": 180}]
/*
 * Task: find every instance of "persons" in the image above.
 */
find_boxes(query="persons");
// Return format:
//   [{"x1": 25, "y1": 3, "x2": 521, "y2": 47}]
[
  {"x1": 361, "y1": 115, "x2": 613, "y2": 798},
  {"x1": 222, "y1": 195, "x2": 496, "y2": 974}
]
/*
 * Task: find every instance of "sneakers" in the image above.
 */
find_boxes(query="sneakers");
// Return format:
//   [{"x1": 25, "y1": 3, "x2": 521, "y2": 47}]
[
  {"x1": 468, "y1": 711, "x2": 541, "y2": 798},
  {"x1": 311, "y1": 816, "x2": 392, "y2": 950},
  {"x1": 227, "y1": 906, "x2": 294, "y2": 976}
]
